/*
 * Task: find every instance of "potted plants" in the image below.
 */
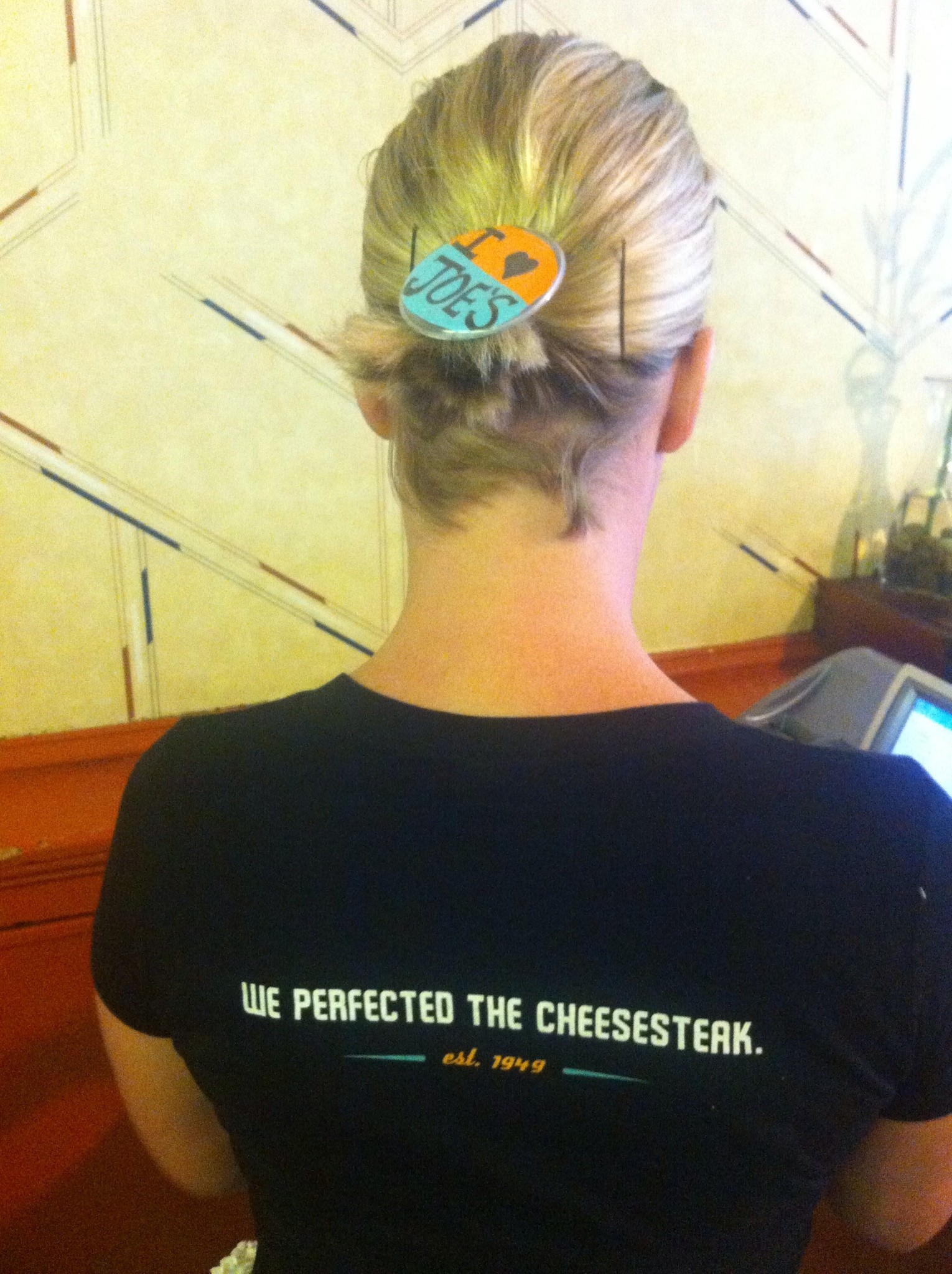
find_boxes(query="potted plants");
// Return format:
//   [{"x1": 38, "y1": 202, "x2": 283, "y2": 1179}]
[{"x1": 809, "y1": 519, "x2": 952, "y2": 681}]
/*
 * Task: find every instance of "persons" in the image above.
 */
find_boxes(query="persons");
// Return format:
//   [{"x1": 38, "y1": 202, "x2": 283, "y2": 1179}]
[{"x1": 85, "y1": 28, "x2": 952, "y2": 1274}]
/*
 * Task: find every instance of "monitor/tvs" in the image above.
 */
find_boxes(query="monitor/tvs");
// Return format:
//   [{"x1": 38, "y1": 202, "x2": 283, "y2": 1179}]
[{"x1": 856, "y1": 664, "x2": 952, "y2": 797}]
